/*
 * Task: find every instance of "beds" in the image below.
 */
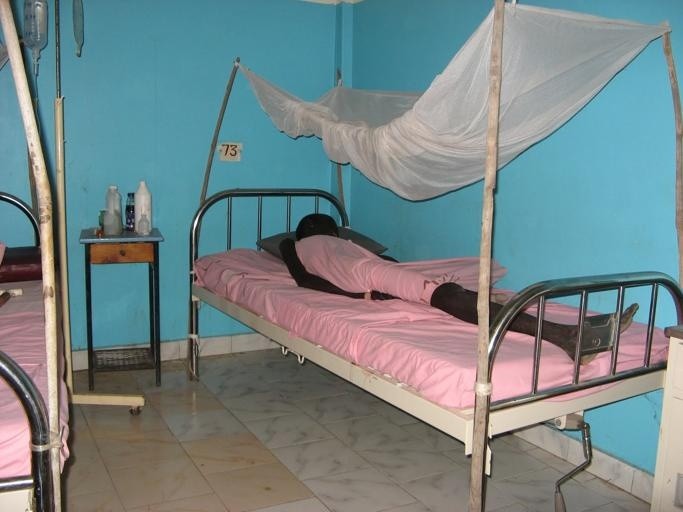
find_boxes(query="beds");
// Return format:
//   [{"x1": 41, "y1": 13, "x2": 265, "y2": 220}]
[
  {"x1": 0, "y1": 189, "x2": 73, "y2": 512},
  {"x1": 185, "y1": 187, "x2": 683, "y2": 512}
]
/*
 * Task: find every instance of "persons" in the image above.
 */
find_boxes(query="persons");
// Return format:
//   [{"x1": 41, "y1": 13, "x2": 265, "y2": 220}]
[{"x1": 280, "y1": 214, "x2": 638, "y2": 367}]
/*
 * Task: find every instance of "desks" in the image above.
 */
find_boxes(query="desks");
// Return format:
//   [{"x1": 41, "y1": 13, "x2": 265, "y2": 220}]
[{"x1": 79, "y1": 228, "x2": 164, "y2": 390}]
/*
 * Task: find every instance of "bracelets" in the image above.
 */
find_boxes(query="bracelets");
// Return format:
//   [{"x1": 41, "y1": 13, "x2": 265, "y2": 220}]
[{"x1": 365, "y1": 292, "x2": 372, "y2": 300}]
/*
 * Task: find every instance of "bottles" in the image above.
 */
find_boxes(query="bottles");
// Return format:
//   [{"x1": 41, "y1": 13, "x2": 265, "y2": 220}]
[
  {"x1": 95, "y1": 211, "x2": 105, "y2": 238},
  {"x1": 22, "y1": 4, "x2": 47, "y2": 61},
  {"x1": 125, "y1": 193, "x2": 149, "y2": 237}
]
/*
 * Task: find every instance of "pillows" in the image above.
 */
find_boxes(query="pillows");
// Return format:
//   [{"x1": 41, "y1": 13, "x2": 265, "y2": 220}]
[{"x1": 254, "y1": 224, "x2": 388, "y2": 262}]
